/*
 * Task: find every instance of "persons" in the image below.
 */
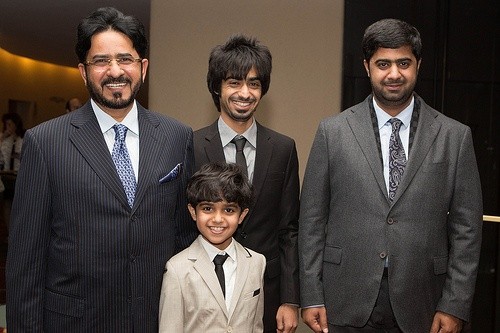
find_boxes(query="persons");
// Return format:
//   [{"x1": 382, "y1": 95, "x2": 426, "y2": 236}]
[
  {"x1": 298, "y1": 19, "x2": 483, "y2": 333},
  {"x1": 0, "y1": 113, "x2": 25, "y2": 243},
  {"x1": 159, "y1": 162, "x2": 267, "y2": 333},
  {"x1": 193, "y1": 35, "x2": 299, "y2": 333},
  {"x1": 5, "y1": 6, "x2": 195, "y2": 333},
  {"x1": 66, "y1": 98, "x2": 83, "y2": 114}
]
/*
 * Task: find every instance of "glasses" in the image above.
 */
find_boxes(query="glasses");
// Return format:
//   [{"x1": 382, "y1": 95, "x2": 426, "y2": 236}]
[{"x1": 85, "y1": 58, "x2": 141, "y2": 69}]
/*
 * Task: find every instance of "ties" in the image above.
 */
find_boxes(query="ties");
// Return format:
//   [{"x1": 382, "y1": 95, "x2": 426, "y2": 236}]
[
  {"x1": 230, "y1": 137, "x2": 249, "y2": 183},
  {"x1": 212, "y1": 254, "x2": 229, "y2": 297},
  {"x1": 388, "y1": 117, "x2": 410, "y2": 206},
  {"x1": 110, "y1": 125, "x2": 139, "y2": 210}
]
